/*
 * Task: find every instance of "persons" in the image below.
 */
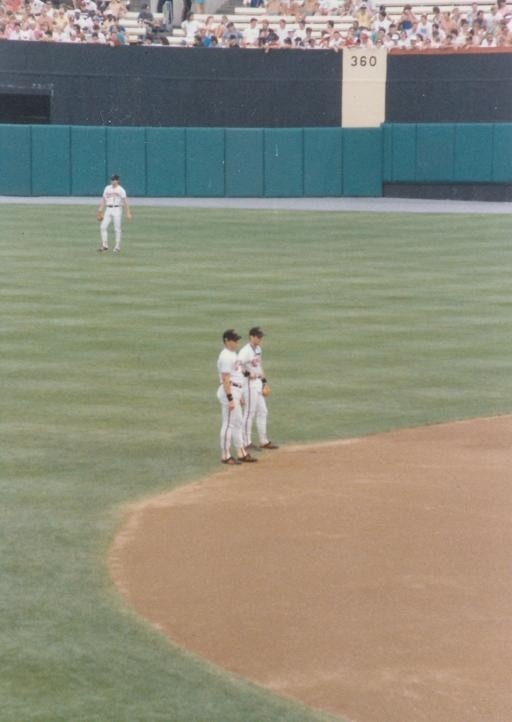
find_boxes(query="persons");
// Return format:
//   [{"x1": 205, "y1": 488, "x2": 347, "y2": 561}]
[
  {"x1": 237, "y1": 326, "x2": 279, "y2": 450},
  {"x1": 215, "y1": 329, "x2": 259, "y2": 467},
  {"x1": 2, "y1": 0, "x2": 512, "y2": 52},
  {"x1": 95, "y1": 175, "x2": 134, "y2": 255}
]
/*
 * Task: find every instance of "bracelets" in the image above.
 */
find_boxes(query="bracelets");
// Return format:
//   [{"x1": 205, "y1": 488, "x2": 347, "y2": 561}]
[
  {"x1": 262, "y1": 378, "x2": 267, "y2": 383},
  {"x1": 226, "y1": 393, "x2": 233, "y2": 402},
  {"x1": 243, "y1": 371, "x2": 250, "y2": 376}
]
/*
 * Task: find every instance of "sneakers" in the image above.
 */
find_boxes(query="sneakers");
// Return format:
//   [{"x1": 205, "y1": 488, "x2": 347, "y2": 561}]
[
  {"x1": 113, "y1": 248, "x2": 120, "y2": 253},
  {"x1": 244, "y1": 443, "x2": 262, "y2": 453},
  {"x1": 222, "y1": 456, "x2": 241, "y2": 465},
  {"x1": 237, "y1": 454, "x2": 257, "y2": 463},
  {"x1": 259, "y1": 441, "x2": 279, "y2": 449},
  {"x1": 98, "y1": 246, "x2": 108, "y2": 252}
]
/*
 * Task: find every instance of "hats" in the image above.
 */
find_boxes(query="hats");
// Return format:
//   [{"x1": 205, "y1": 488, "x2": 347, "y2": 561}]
[
  {"x1": 249, "y1": 327, "x2": 266, "y2": 337},
  {"x1": 223, "y1": 329, "x2": 241, "y2": 342},
  {"x1": 111, "y1": 173, "x2": 118, "y2": 180}
]
